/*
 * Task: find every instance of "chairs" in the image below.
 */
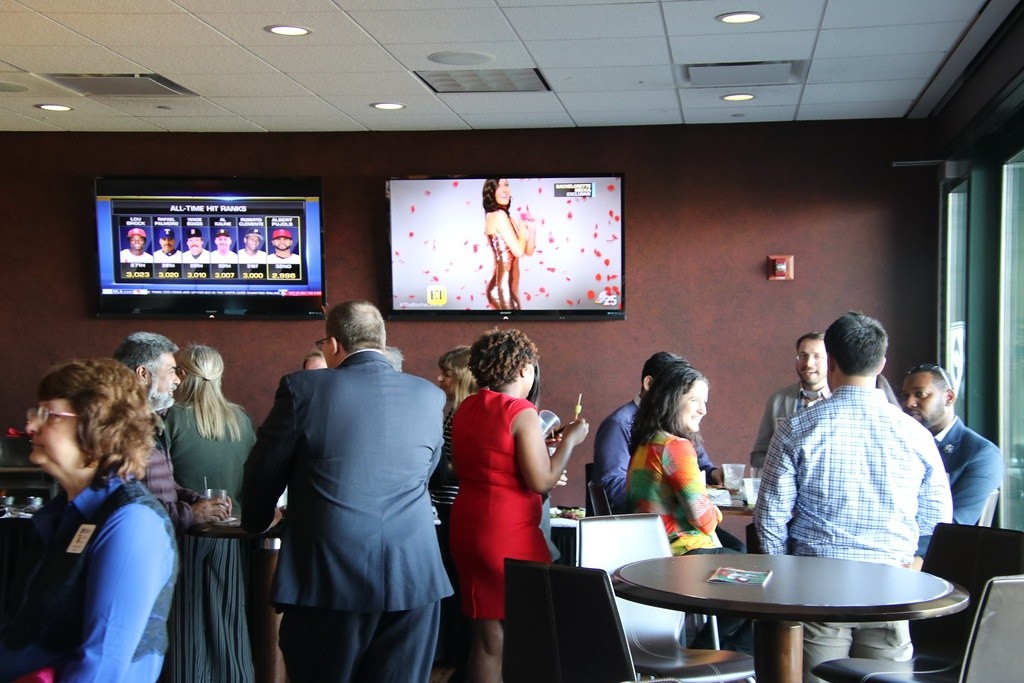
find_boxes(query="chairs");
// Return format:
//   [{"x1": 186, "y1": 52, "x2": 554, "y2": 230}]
[{"x1": 502, "y1": 464, "x2": 1024, "y2": 683}]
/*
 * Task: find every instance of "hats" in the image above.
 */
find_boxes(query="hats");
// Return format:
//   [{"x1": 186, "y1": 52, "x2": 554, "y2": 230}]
[
  {"x1": 216, "y1": 229, "x2": 231, "y2": 237},
  {"x1": 126, "y1": 228, "x2": 147, "y2": 238},
  {"x1": 244, "y1": 228, "x2": 263, "y2": 240},
  {"x1": 159, "y1": 226, "x2": 175, "y2": 239},
  {"x1": 186, "y1": 228, "x2": 202, "y2": 238},
  {"x1": 272, "y1": 228, "x2": 292, "y2": 239}
]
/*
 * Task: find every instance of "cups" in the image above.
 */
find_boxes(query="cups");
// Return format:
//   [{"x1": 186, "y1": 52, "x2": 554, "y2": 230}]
[
  {"x1": 205, "y1": 489, "x2": 226, "y2": 502},
  {"x1": 538, "y1": 409, "x2": 561, "y2": 439},
  {"x1": 722, "y1": 463, "x2": 745, "y2": 490},
  {"x1": 743, "y1": 477, "x2": 761, "y2": 504}
]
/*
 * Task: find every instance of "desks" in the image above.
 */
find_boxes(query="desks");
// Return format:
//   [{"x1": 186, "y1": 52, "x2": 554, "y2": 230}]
[
  {"x1": 186, "y1": 515, "x2": 287, "y2": 683},
  {"x1": 718, "y1": 490, "x2": 755, "y2": 515},
  {"x1": 610, "y1": 553, "x2": 970, "y2": 683}
]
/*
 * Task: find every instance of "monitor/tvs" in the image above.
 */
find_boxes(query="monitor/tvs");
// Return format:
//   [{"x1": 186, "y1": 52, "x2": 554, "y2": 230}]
[
  {"x1": 378, "y1": 171, "x2": 627, "y2": 321},
  {"x1": 75, "y1": 175, "x2": 327, "y2": 321}
]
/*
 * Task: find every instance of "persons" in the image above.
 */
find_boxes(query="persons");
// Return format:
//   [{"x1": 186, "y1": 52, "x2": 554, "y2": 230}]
[
  {"x1": 120, "y1": 227, "x2": 153, "y2": 263},
  {"x1": 182, "y1": 228, "x2": 209, "y2": 263},
  {"x1": 594, "y1": 351, "x2": 725, "y2": 511},
  {"x1": 154, "y1": 227, "x2": 182, "y2": 262},
  {"x1": 750, "y1": 333, "x2": 832, "y2": 467},
  {"x1": 211, "y1": 229, "x2": 237, "y2": 263},
  {"x1": 0, "y1": 358, "x2": 180, "y2": 683},
  {"x1": 754, "y1": 310, "x2": 953, "y2": 683},
  {"x1": 238, "y1": 228, "x2": 266, "y2": 263},
  {"x1": 483, "y1": 179, "x2": 535, "y2": 311},
  {"x1": 626, "y1": 362, "x2": 742, "y2": 557},
  {"x1": 268, "y1": 229, "x2": 299, "y2": 264},
  {"x1": 239, "y1": 298, "x2": 590, "y2": 683},
  {"x1": 902, "y1": 364, "x2": 1003, "y2": 571},
  {"x1": 156, "y1": 344, "x2": 257, "y2": 683},
  {"x1": 112, "y1": 332, "x2": 231, "y2": 536}
]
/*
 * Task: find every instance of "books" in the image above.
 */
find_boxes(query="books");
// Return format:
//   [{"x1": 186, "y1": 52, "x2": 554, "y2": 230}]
[{"x1": 708, "y1": 567, "x2": 773, "y2": 587}]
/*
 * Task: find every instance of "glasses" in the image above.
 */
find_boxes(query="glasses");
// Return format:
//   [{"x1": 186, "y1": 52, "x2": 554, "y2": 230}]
[
  {"x1": 26, "y1": 406, "x2": 80, "y2": 424},
  {"x1": 795, "y1": 352, "x2": 824, "y2": 361},
  {"x1": 316, "y1": 335, "x2": 339, "y2": 350},
  {"x1": 905, "y1": 363, "x2": 952, "y2": 390}
]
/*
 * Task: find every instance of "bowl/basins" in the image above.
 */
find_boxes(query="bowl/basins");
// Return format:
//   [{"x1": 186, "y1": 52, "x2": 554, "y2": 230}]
[
  {"x1": 25, "y1": 496, "x2": 43, "y2": 506},
  {"x1": 2, "y1": 496, "x2": 14, "y2": 506}
]
